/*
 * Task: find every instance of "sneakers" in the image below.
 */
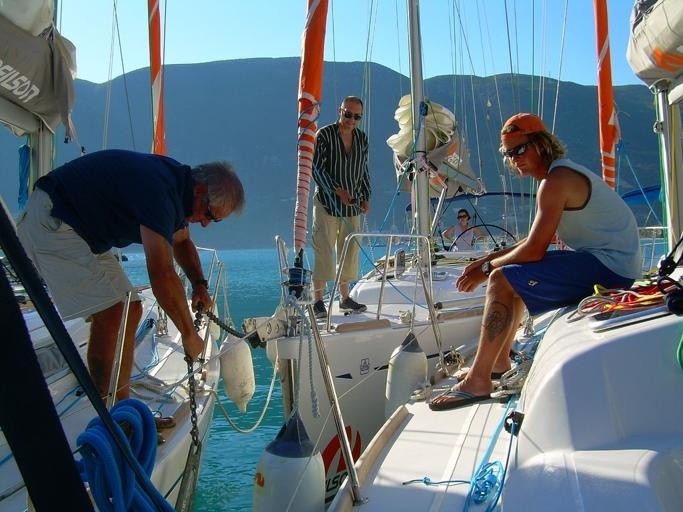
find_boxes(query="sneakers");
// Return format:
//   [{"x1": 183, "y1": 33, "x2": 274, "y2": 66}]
[
  {"x1": 313, "y1": 300, "x2": 327, "y2": 318},
  {"x1": 338, "y1": 296, "x2": 367, "y2": 313}
]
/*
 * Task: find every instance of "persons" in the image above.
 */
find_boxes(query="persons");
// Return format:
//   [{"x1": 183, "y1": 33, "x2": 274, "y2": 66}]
[
  {"x1": 441, "y1": 208, "x2": 486, "y2": 251},
  {"x1": 310, "y1": 95, "x2": 372, "y2": 319},
  {"x1": 15, "y1": 148, "x2": 245, "y2": 444},
  {"x1": 428, "y1": 111, "x2": 644, "y2": 410}
]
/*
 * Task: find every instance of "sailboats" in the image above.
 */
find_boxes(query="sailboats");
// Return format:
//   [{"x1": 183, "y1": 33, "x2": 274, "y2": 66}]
[
  {"x1": 245, "y1": 1, "x2": 525, "y2": 512},
  {"x1": 328, "y1": 0, "x2": 683, "y2": 512},
  {"x1": 0, "y1": 1, "x2": 220, "y2": 508}
]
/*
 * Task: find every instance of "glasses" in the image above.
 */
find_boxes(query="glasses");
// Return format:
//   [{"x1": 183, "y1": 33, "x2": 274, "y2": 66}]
[
  {"x1": 204, "y1": 179, "x2": 223, "y2": 223},
  {"x1": 503, "y1": 140, "x2": 531, "y2": 157},
  {"x1": 341, "y1": 108, "x2": 361, "y2": 121},
  {"x1": 458, "y1": 215, "x2": 466, "y2": 218}
]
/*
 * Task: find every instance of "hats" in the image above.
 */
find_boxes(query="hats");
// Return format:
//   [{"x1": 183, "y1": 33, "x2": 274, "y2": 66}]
[{"x1": 500, "y1": 111, "x2": 546, "y2": 141}]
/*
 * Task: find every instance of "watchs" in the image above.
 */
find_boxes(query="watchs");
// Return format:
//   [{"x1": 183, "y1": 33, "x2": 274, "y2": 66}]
[
  {"x1": 192, "y1": 279, "x2": 209, "y2": 289},
  {"x1": 481, "y1": 260, "x2": 491, "y2": 276}
]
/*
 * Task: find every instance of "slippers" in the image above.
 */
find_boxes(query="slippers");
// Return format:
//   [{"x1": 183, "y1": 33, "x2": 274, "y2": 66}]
[
  {"x1": 429, "y1": 387, "x2": 490, "y2": 411},
  {"x1": 457, "y1": 368, "x2": 513, "y2": 383}
]
